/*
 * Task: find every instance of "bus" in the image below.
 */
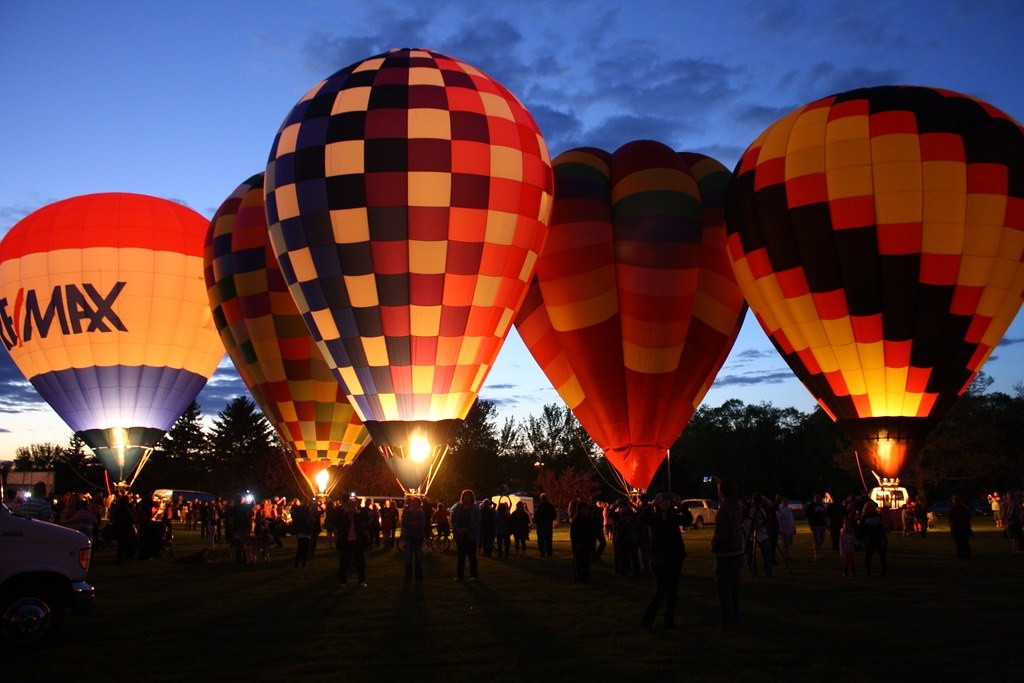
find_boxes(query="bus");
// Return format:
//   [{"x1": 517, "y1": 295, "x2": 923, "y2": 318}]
[
  {"x1": 152, "y1": 489, "x2": 218, "y2": 506},
  {"x1": 356, "y1": 495, "x2": 405, "y2": 522}
]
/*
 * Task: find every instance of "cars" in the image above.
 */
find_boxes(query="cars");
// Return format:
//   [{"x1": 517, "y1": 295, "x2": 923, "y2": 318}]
[
  {"x1": 0, "y1": 498, "x2": 96, "y2": 651},
  {"x1": 930, "y1": 500, "x2": 951, "y2": 517},
  {"x1": 681, "y1": 498, "x2": 721, "y2": 530},
  {"x1": 973, "y1": 504, "x2": 992, "y2": 516}
]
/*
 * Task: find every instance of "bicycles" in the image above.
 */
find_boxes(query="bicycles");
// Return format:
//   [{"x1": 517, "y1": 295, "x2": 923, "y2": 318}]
[{"x1": 396, "y1": 523, "x2": 451, "y2": 554}]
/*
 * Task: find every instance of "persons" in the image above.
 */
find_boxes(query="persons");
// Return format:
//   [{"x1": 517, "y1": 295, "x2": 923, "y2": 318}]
[
  {"x1": 711, "y1": 481, "x2": 746, "y2": 631},
  {"x1": 3, "y1": 481, "x2": 1024, "y2": 588},
  {"x1": 640, "y1": 491, "x2": 693, "y2": 636}
]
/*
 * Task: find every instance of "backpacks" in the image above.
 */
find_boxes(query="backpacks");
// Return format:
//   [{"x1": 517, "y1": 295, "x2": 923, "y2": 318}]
[{"x1": 455, "y1": 506, "x2": 475, "y2": 529}]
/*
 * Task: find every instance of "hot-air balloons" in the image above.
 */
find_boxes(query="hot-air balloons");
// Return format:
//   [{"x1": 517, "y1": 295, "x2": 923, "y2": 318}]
[
  {"x1": 510, "y1": 139, "x2": 749, "y2": 511},
  {"x1": 263, "y1": 46, "x2": 556, "y2": 504},
  {"x1": 203, "y1": 169, "x2": 372, "y2": 536},
  {"x1": 0, "y1": 191, "x2": 226, "y2": 531},
  {"x1": 722, "y1": 84, "x2": 1024, "y2": 530}
]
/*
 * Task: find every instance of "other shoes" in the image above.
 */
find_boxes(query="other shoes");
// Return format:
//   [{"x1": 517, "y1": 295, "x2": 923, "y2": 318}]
[
  {"x1": 452, "y1": 577, "x2": 464, "y2": 582},
  {"x1": 340, "y1": 583, "x2": 346, "y2": 588},
  {"x1": 360, "y1": 582, "x2": 368, "y2": 588},
  {"x1": 664, "y1": 623, "x2": 680, "y2": 630},
  {"x1": 640, "y1": 625, "x2": 657, "y2": 633},
  {"x1": 469, "y1": 576, "x2": 480, "y2": 582}
]
